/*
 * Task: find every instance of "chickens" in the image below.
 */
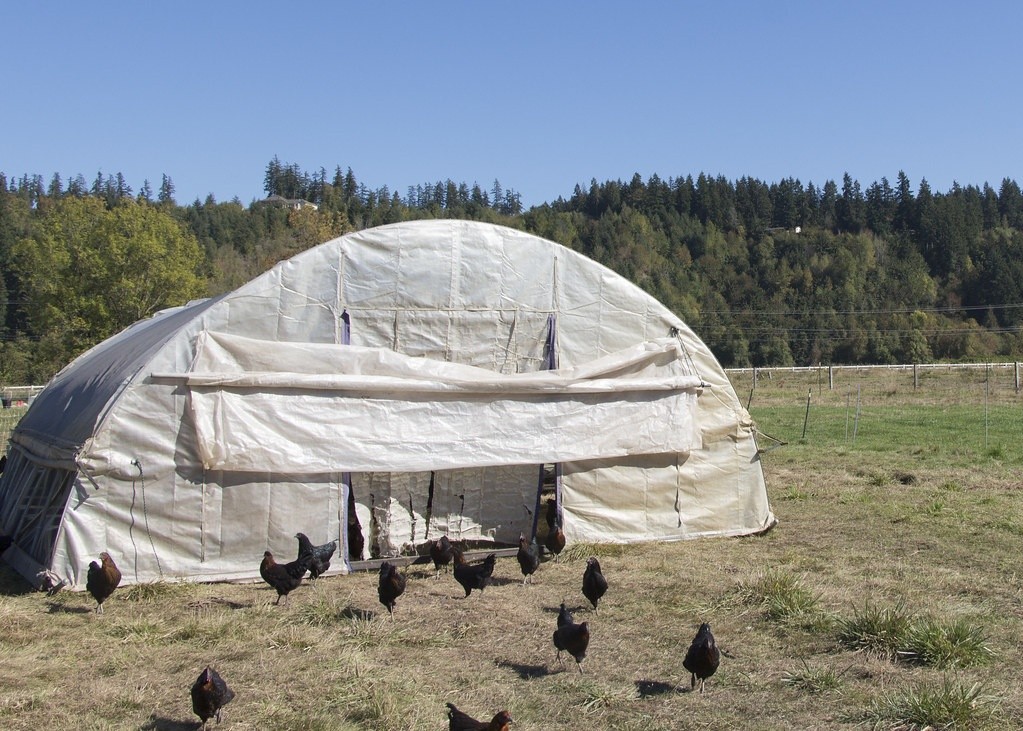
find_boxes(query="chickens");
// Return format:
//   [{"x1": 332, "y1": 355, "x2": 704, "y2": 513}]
[
  {"x1": 349, "y1": 524, "x2": 362, "y2": 560},
  {"x1": 294, "y1": 533, "x2": 337, "y2": 586},
  {"x1": 259, "y1": 550, "x2": 313, "y2": 604},
  {"x1": 546, "y1": 499, "x2": 557, "y2": 526},
  {"x1": 554, "y1": 603, "x2": 589, "y2": 675},
  {"x1": 682, "y1": 620, "x2": 719, "y2": 693},
  {"x1": 86, "y1": 552, "x2": 122, "y2": 614},
  {"x1": 545, "y1": 526, "x2": 566, "y2": 564},
  {"x1": 377, "y1": 559, "x2": 406, "y2": 624},
  {"x1": 581, "y1": 557, "x2": 609, "y2": 617},
  {"x1": 191, "y1": 664, "x2": 235, "y2": 731},
  {"x1": 445, "y1": 703, "x2": 514, "y2": 731},
  {"x1": 429, "y1": 538, "x2": 454, "y2": 581},
  {"x1": 516, "y1": 531, "x2": 540, "y2": 586},
  {"x1": 447, "y1": 546, "x2": 497, "y2": 599}
]
